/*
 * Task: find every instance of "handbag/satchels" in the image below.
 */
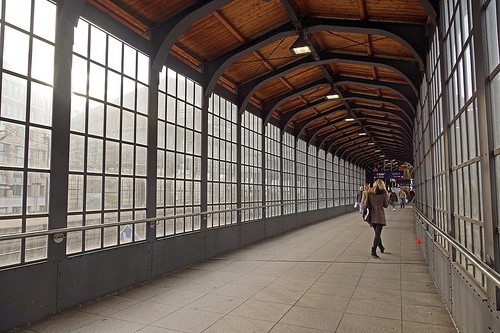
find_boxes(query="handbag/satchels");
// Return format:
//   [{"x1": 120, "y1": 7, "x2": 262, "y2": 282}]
[
  {"x1": 362, "y1": 194, "x2": 372, "y2": 223},
  {"x1": 354, "y1": 202, "x2": 357, "y2": 208}
]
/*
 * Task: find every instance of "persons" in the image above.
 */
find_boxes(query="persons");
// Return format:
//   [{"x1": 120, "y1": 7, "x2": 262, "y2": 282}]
[
  {"x1": 403, "y1": 188, "x2": 415, "y2": 204},
  {"x1": 366, "y1": 179, "x2": 389, "y2": 258},
  {"x1": 390, "y1": 189, "x2": 398, "y2": 211},
  {"x1": 398, "y1": 188, "x2": 406, "y2": 208},
  {"x1": 357, "y1": 185, "x2": 374, "y2": 221}
]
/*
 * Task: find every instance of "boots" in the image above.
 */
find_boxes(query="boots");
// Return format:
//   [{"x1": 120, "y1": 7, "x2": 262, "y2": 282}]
[
  {"x1": 371, "y1": 246, "x2": 379, "y2": 258},
  {"x1": 378, "y1": 242, "x2": 385, "y2": 253}
]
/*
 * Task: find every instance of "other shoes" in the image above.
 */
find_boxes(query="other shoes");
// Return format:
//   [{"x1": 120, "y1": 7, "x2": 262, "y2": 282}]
[{"x1": 393, "y1": 208, "x2": 395, "y2": 211}]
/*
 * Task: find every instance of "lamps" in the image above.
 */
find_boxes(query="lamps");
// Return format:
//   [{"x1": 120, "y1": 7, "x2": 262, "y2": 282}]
[
  {"x1": 289, "y1": 35, "x2": 311, "y2": 55},
  {"x1": 325, "y1": 87, "x2": 339, "y2": 100},
  {"x1": 345, "y1": 110, "x2": 354, "y2": 121}
]
